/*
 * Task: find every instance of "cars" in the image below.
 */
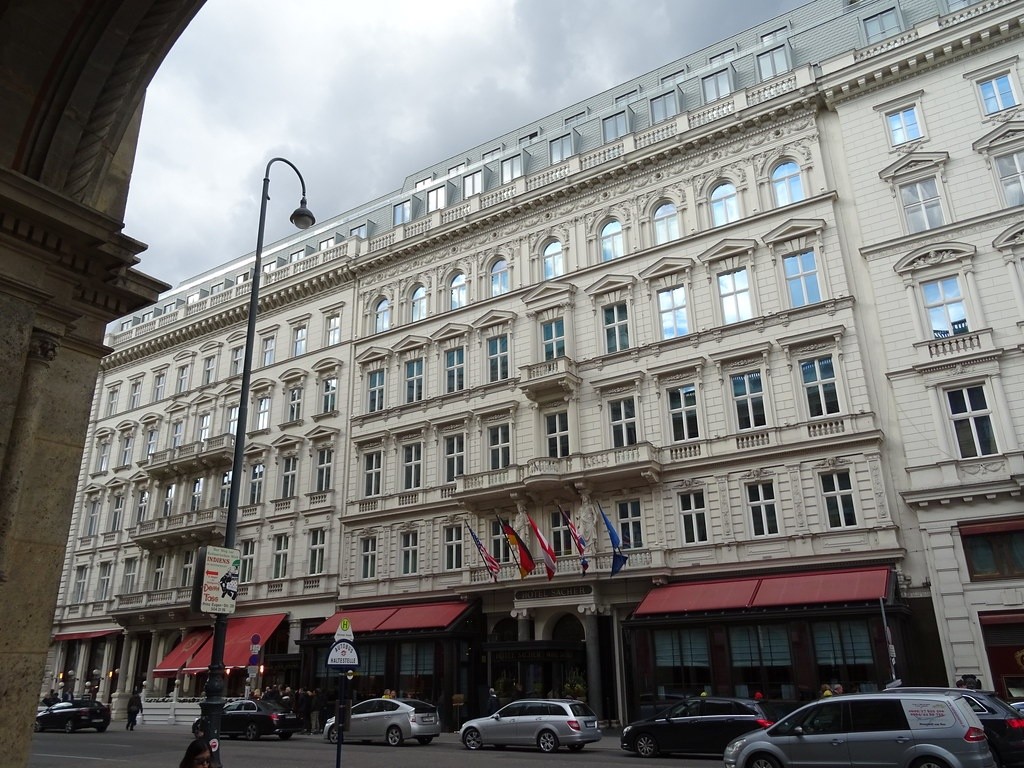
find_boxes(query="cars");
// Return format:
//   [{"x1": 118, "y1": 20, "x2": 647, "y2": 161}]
[
  {"x1": 460, "y1": 697, "x2": 603, "y2": 753},
  {"x1": 192, "y1": 700, "x2": 303, "y2": 743},
  {"x1": 638, "y1": 693, "x2": 693, "y2": 724},
  {"x1": 620, "y1": 696, "x2": 784, "y2": 757},
  {"x1": 36, "y1": 702, "x2": 48, "y2": 713},
  {"x1": 808, "y1": 688, "x2": 1024, "y2": 768},
  {"x1": 35, "y1": 698, "x2": 111, "y2": 732},
  {"x1": 325, "y1": 695, "x2": 442, "y2": 747},
  {"x1": 723, "y1": 691, "x2": 999, "y2": 768}
]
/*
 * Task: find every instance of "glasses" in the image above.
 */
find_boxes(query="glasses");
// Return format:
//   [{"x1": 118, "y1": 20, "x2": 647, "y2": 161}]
[{"x1": 193, "y1": 757, "x2": 212, "y2": 765}]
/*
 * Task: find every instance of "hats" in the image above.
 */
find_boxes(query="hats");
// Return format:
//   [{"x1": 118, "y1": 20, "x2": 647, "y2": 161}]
[{"x1": 956, "y1": 680, "x2": 967, "y2": 687}]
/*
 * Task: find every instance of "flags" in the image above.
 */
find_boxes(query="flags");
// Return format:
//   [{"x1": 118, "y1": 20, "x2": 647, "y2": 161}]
[{"x1": 464, "y1": 500, "x2": 629, "y2": 578}]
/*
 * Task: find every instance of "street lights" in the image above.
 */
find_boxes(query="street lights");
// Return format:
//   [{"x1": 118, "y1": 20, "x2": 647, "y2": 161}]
[
  {"x1": 86, "y1": 670, "x2": 102, "y2": 702},
  {"x1": 185, "y1": 158, "x2": 316, "y2": 762},
  {"x1": 58, "y1": 669, "x2": 75, "y2": 699}
]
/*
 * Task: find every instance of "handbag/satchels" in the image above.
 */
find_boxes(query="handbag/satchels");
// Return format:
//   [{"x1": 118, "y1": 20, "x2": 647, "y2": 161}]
[
  {"x1": 130, "y1": 705, "x2": 139, "y2": 712},
  {"x1": 42, "y1": 698, "x2": 49, "y2": 706}
]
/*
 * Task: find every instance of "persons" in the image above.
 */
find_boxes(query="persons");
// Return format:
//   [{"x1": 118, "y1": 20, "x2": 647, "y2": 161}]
[
  {"x1": 382, "y1": 689, "x2": 396, "y2": 699},
  {"x1": 62, "y1": 688, "x2": 74, "y2": 700},
  {"x1": 956, "y1": 675, "x2": 982, "y2": 690},
  {"x1": 42, "y1": 689, "x2": 61, "y2": 707},
  {"x1": 126, "y1": 691, "x2": 143, "y2": 731},
  {"x1": 179, "y1": 739, "x2": 212, "y2": 768},
  {"x1": 818, "y1": 683, "x2": 843, "y2": 698},
  {"x1": 249, "y1": 685, "x2": 327, "y2": 735}
]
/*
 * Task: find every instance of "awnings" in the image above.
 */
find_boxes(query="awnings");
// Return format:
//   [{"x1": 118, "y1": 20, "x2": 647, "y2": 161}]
[
  {"x1": 633, "y1": 567, "x2": 890, "y2": 615},
  {"x1": 308, "y1": 600, "x2": 472, "y2": 635},
  {"x1": 152, "y1": 613, "x2": 287, "y2": 678},
  {"x1": 48, "y1": 628, "x2": 123, "y2": 645}
]
